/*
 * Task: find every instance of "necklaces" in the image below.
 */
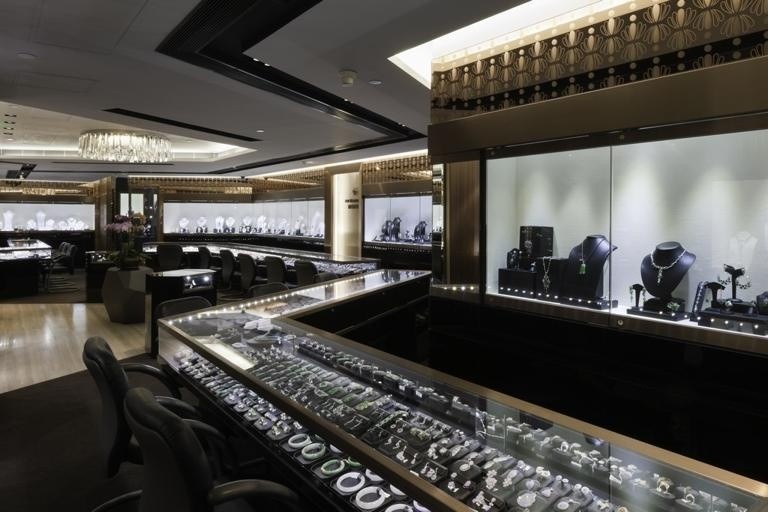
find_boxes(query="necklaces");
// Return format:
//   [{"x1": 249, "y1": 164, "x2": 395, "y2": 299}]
[
  {"x1": 650, "y1": 249, "x2": 689, "y2": 286},
  {"x1": 540, "y1": 255, "x2": 554, "y2": 292},
  {"x1": 579, "y1": 239, "x2": 604, "y2": 276}
]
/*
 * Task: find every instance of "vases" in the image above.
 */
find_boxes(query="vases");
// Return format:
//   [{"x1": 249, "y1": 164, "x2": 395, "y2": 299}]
[{"x1": 120, "y1": 255, "x2": 140, "y2": 271}]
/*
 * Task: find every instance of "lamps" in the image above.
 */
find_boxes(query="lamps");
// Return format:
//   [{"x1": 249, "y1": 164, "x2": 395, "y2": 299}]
[{"x1": 77, "y1": 128, "x2": 174, "y2": 165}]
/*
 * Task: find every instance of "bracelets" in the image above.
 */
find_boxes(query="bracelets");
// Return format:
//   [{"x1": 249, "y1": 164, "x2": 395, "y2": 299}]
[{"x1": 288, "y1": 430, "x2": 435, "y2": 511}]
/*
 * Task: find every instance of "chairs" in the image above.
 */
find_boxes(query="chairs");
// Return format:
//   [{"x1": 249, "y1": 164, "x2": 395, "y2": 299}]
[
  {"x1": 38, "y1": 242, "x2": 80, "y2": 294},
  {"x1": 154, "y1": 241, "x2": 339, "y2": 299},
  {"x1": 93, "y1": 389, "x2": 308, "y2": 512},
  {"x1": 153, "y1": 296, "x2": 212, "y2": 352},
  {"x1": 82, "y1": 337, "x2": 218, "y2": 488}
]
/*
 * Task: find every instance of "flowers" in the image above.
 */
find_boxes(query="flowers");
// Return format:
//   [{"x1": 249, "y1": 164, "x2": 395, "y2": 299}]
[{"x1": 104, "y1": 211, "x2": 152, "y2": 242}]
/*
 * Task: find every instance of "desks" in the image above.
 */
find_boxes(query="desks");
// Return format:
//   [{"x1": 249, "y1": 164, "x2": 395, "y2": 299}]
[{"x1": 101, "y1": 266, "x2": 154, "y2": 324}]
